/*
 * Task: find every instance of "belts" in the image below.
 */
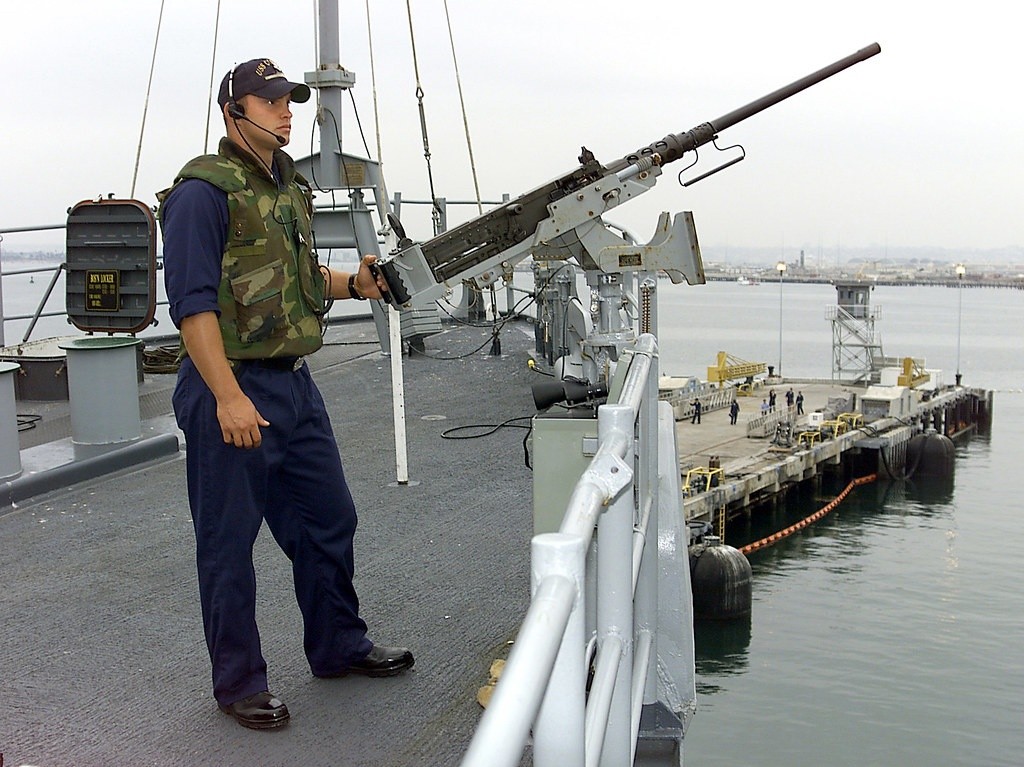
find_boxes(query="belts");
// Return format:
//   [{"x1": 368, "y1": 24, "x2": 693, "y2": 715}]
[{"x1": 244, "y1": 354, "x2": 308, "y2": 371}]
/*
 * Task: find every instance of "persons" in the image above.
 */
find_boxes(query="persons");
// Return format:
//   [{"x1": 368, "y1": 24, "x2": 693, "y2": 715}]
[
  {"x1": 689, "y1": 398, "x2": 701, "y2": 424},
  {"x1": 156, "y1": 59, "x2": 415, "y2": 729},
  {"x1": 785, "y1": 388, "x2": 794, "y2": 406},
  {"x1": 796, "y1": 391, "x2": 804, "y2": 415},
  {"x1": 761, "y1": 399, "x2": 770, "y2": 416},
  {"x1": 730, "y1": 399, "x2": 740, "y2": 425},
  {"x1": 769, "y1": 389, "x2": 776, "y2": 413}
]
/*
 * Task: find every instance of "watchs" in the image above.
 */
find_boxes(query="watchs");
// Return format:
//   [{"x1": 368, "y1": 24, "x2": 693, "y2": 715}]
[{"x1": 348, "y1": 272, "x2": 366, "y2": 300}]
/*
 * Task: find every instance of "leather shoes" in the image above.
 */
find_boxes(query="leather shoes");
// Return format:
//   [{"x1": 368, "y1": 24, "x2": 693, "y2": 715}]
[
  {"x1": 322, "y1": 644, "x2": 414, "y2": 681},
  {"x1": 217, "y1": 691, "x2": 291, "y2": 730}
]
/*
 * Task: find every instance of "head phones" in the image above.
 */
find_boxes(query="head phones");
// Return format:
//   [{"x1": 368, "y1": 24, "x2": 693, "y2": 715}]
[{"x1": 227, "y1": 64, "x2": 244, "y2": 120}]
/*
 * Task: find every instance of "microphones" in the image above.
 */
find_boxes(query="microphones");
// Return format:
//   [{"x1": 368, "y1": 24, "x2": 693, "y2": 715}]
[{"x1": 231, "y1": 109, "x2": 286, "y2": 143}]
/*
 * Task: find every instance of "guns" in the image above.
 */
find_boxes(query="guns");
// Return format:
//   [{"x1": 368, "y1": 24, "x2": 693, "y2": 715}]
[{"x1": 368, "y1": 42, "x2": 882, "y2": 312}]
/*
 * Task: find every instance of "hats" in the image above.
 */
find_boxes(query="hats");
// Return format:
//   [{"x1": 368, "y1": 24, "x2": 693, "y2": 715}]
[{"x1": 218, "y1": 58, "x2": 311, "y2": 111}]
